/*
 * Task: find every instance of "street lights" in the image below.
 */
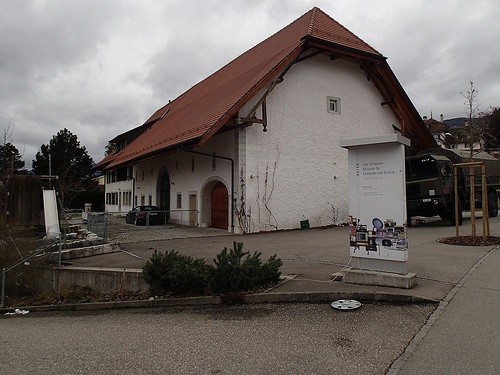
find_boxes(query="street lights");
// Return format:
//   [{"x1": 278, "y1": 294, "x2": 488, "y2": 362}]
[{"x1": 10, "y1": 151, "x2": 15, "y2": 175}]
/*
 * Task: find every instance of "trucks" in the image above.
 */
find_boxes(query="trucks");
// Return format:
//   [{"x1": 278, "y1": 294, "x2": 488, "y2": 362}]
[{"x1": 404, "y1": 147, "x2": 500, "y2": 225}]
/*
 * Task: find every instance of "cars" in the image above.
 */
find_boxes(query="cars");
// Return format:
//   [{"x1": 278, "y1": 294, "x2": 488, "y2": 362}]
[{"x1": 124, "y1": 206, "x2": 170, "y2": 226}]
